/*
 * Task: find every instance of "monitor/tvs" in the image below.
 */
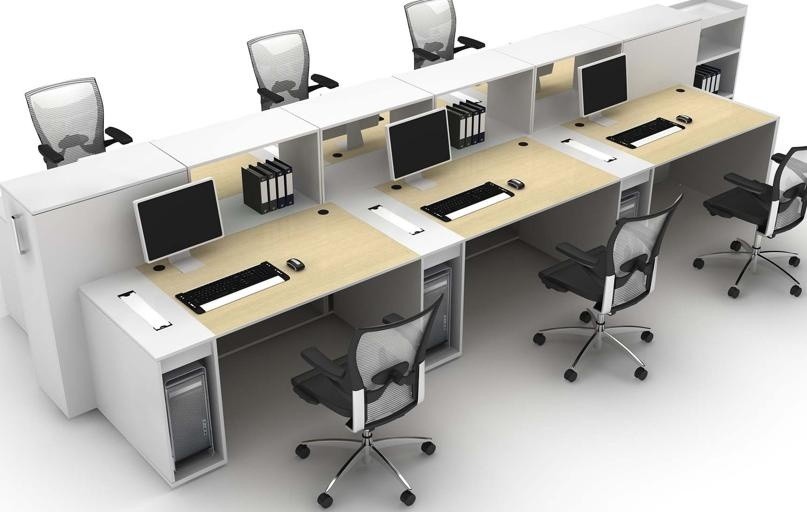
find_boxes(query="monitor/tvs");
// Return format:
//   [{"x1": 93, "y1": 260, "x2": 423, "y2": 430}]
[
  {"x1": 322, "y1": 115, "x2": 379, "y2": 151},
  {"x1": 577, "y1": 53, "x2": 627, "y2": 127},
  {"x1": 536, "y1": 63, "x2": 554, "y2": 94},
  {"x1": 385, "y1": 106, "x2": 452, "y2": 191},
  {"x1": 132, "y1": 176, "x2": 224, "y2": 274}
]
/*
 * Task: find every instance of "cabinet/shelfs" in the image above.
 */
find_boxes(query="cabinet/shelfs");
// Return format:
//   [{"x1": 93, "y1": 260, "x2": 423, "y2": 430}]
[
  {"x1": 672, "y1": 0, "x2": 753, "y2": 100},
  {"x1": 1, "y1": 139, "x2": 193, "y2": 419}
]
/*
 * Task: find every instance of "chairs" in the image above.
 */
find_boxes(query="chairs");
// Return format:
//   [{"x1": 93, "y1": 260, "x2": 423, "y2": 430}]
[
  {"x1": 527, "y1": 183, "x2": 684, "y2": 385},
  {"x1": 21, "y1": 74, "x2": 134, "y2": 170},
  {"x1": 689, "y1": 136, "x2": 806, "y2": 304},
  {"x1": 402, "y1": 1, "x2": 488, "y2": 70},
  {"x1": 244, "y1": 26, "x2": 337, "y2": 105},
  {"x1": 284, "y1": 288, "x2": 444, "y2": 510}
]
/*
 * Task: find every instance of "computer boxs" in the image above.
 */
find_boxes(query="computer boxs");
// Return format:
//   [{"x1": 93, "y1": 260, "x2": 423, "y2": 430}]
[
  {"x1": 162, "y1": 361, "x2": 214, "y2": 472},
  {"x1": 423, "y1": 264, "x2": 453, "y2": 356},
  {"x1": 620, "y1": 190, "x2": 640, "y2": 224}
]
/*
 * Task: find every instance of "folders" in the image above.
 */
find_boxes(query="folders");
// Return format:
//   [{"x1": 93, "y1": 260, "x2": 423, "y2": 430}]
[
  {"x1": 694, "y1": 64, "x2": 721, "y2": 94},
  {"x1": 446, "y1": 100, "x2": 486, "y2": 149},
  {"x1": 241, "y1": 157, "x2": 295, "y2": 215}
]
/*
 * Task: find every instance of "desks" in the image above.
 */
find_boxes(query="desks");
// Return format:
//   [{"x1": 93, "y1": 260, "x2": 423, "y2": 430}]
[
  {"x1": 453, "y1": 51, "x2": 585, "y2": 104},
  {"x1": 319, "y1": 92, "x2": 462, "y2": 164},
  {"x1": 565, "y1": 80, "x2": 781, "y2": 292},
  {"x1": 174, "y1": 128, "x2": 275, "y2": 199},
  {"x1": 82, "y1": 182, "x2": 465, "y2": 492},
  {"x1": 385, "y1": 139, "x2": 658, "y2": 284}
]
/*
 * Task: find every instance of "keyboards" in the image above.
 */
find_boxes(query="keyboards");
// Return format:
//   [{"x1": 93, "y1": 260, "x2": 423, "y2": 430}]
[
  {"x1": 421, "y1": 181, "x2": 514, "y2": 222},
  {"x1": 606, "y1": 117, "x2": 685, "y2": 149},
  {"x1": 174, "y1": 261, "x2": 290, "y2": 314}
]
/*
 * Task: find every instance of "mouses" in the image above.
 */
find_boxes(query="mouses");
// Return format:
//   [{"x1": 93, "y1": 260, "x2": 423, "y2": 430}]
[
  {"x1": 287, "y1": 258, "x2": 305, "y2": 269},
  {"x1": 508, "y1": 178, "x2": 525, "y2": 190},
  {"x1": 676, "y1": 114, "x2": 692, "y2": 123}
]
